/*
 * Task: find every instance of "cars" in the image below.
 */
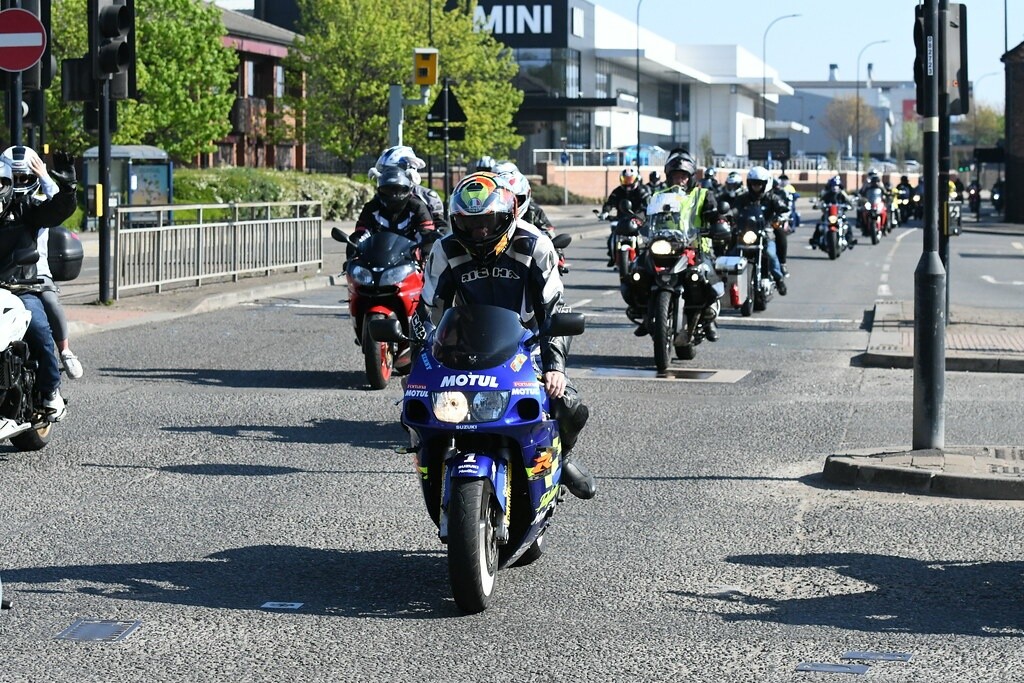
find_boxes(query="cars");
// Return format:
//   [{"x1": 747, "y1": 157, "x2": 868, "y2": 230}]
[
  {"x1": 786, "y1": 153, "x2": 923, "y2": 172},
  {"x1": 603, "y1": 144, "x2": 663, "y2": 163}
]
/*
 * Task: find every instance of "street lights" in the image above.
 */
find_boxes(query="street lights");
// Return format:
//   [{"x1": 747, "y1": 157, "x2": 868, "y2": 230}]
[
  {"x1": 856, "y1": 38, "x2": 890, "y2": 195},
  {"x1": 764, "y1": 13, "x2": 804, "y2": 137},
  {"x1": 664, "y1": 71, "x2": 682, "y2": 149}
]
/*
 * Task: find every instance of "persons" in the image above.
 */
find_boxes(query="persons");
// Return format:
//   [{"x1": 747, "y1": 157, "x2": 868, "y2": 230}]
[
  {"x1": 342, "y1": 145, "x2": 449, "y2": 268},
  {"x1": 400, "y1": 171, "x2": 599, "y2": 501},
  {"x1": 477, "y1": 155, "x2": 518, "y2": 176},
  {"x1": 948, "y1": 169, "x2": 981, "y2": 212},
  {"x1": 991, "y1": 178, "x2": 1004, "y2": 205},
  {"x1": 810, "y1": 169, "x2": 925, "y2": 250},
  {"x1": 598, "y1": 150, "x2": 798, "y2": 343},
  {"x1": 0, "y1": 145, "x2": 84, "y2": 422}
]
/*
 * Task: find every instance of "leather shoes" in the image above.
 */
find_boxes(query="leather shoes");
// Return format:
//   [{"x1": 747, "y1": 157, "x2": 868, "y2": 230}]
[
  {"x1": 703, "y1": 320, "x2": 720, "y2": 342},
  {"x1": 561, "y1": 450, "x2": 596, "y2": 500}
]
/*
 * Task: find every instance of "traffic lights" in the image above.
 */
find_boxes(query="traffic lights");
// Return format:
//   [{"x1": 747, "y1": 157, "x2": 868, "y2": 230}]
[
  {"x1": 87, "y1": 0, "x2": 134, "y2": 79},
  {"x1": 958, "y1": 161, "x2": 976, "y2": 172}
]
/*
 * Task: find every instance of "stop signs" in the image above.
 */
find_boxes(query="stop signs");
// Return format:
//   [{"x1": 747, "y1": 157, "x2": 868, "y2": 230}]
[{"x1": 0, "y1": 8, "x2": 48, "y2": 73}]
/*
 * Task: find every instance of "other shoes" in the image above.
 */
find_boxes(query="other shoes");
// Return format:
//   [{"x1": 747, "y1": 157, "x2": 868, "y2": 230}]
[
  {"x1": 848, "y1": 239, "x2": 858, "y2": 250},
  {"x1": 775, "y1": 280, "x2": 786, "y2": 296},
  {"x1": 634, "y1": 319, "x2": 653, "y2": 337},
  {"x1": 809, "y1": 239, "x2": 817, "y2": 250}
]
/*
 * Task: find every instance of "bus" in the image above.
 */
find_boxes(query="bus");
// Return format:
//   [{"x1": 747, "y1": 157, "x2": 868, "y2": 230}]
[{"x1": 532, "y1": 148, "x2": 627, "y2": 166}]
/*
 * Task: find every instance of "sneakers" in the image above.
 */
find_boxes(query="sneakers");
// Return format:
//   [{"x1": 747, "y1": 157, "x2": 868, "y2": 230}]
[
  {"x1": 59, "y1": 347, "x2": 84, "y2": 379},
  {"x1": 43, "y1": 387, "x2": 67, "y2": 422}
]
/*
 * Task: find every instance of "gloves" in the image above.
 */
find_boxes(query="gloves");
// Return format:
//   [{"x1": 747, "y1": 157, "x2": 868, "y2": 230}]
[{"x1": 600, "y1": 212, "x2": 608, "y2": 221}]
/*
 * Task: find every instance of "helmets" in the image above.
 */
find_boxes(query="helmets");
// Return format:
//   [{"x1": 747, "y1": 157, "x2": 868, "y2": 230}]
[
  {"x1": 448, "y1": 171, "x2": 518, "y2": 265},
  {"x1": 649, "y1": 171, "x2": 661, "y2": 187},
  {"x1": 725, "y1": 173, "x2": 745, "y2": 197},
  {"x1": 704, "y1": 168, "x2": 715, "y2": 177},
  {"x1": 476, "y1": 155, "x2": 496, "y2": 172},
  {"x1": 665, "y1": 152, "x2": 696, "y2": 189},
  {"x1": 747, "y1": 166, "x2": 773, "y2": 197},
  {"x1": 0, "y1": 160, "x2": 14, "y2": 216},
  {"x1": 619, "y1": 169, "x2": 639, "y2": 192},
  {"x1": 379, "y1": 167, "x2": 411, "y2": 213},
  {"x1": 368, "y1": 146, "x2": 426, "y2": 184},
  {"x1": 773, "y1": 179, "x2": 782, "y2": 195},
  {"x1": 0, "y1": 145, "x2": 41, "y2": 197},
  {"x1": 497, "y1": 171, "x2": 532, "y2": 219},
  {"x1": 491, "y1": 160, "x2": 519, "y2": 175},
  {"x1": 828, "y1": 179, "x2": 841, "y2": 187}
]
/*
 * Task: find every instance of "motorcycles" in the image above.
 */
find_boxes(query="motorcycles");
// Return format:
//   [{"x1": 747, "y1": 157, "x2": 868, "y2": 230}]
[
  {"x1": 331, "y1": 227, "x2": 455, "y2": 389},
  {"x1": 729, "y1": 203, "x2": 797, "y2": 317},
  {"x1": 593, "y1": 197, "x2": 731, "y2": 370},
  {"x1": 366, "y1": 232, "x2": 585, "y2": 615},
  {"x1": 0, "y1": 226, "x2": 85, "y2": 454},
  {"x1": 855, "y1": 188, "x2": 926, "y2": 245},
  {"x1": 950, "y1": 189, "x2": 1007, "y2": 212},
  {"x1": 808, "y1": 195, "x2": 861, "y2": 259}
]
